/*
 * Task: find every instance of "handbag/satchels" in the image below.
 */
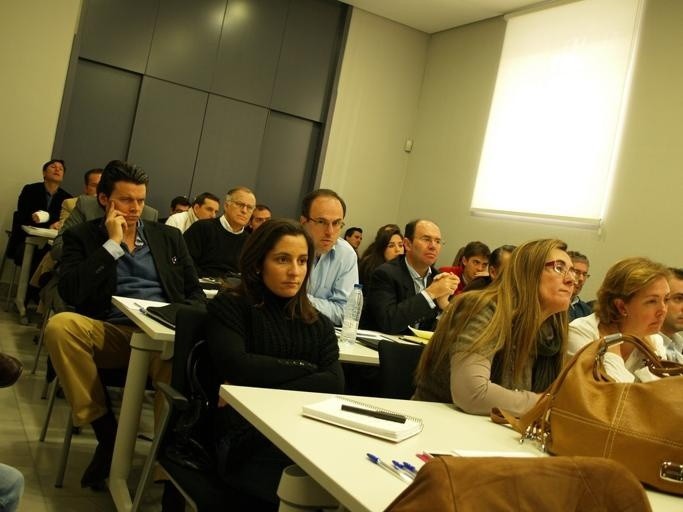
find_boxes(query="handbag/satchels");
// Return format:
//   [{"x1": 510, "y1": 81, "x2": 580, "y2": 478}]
[{"x1": 491, "y1": 333, "x2": 683, "y2": 496}]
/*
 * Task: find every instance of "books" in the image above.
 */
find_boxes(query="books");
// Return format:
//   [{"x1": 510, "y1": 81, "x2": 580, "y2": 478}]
[{"x1": 299, "y1": 393, "x2": 425, "y2": 443}]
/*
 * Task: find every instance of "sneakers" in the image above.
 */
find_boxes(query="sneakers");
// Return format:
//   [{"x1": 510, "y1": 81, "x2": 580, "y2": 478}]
[{"x1": 0, "y1": 353, "x2": 24, "y2": 388}]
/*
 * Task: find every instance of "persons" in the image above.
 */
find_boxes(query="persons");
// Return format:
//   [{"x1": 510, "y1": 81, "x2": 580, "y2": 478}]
[
  {"x1": 183, "y1": 187, "x2": 257, "y2": 280},
  {"x1": 412, "y1": 237, "x2": 579, "y2": 420},
  {"x1": 363, "y1": 220, "x2": 460, "y2": 336},
  {"x1": 4, "y1": 162, "x2": 75, "y2": 317},
  {"x1": 463, "y1": 245, "x2": 517, "y2": 293},
  {"x1": 660, "y1": 267, "x2": 683, "y2": 355},
  {"x1": 169, "y1": 196, "x2": 191, "y2": 216},
  {"x1": 46, "y1": 159, "x2": 207, "y2": 491},
  {"x1": 164, "y1": 192, "x2": 219, "y2": 234},
  {"x1": 155, "y1": 215, "x2": 348, "y2": 509},
  {"x1": 249, "y1": 205, "x2": 273, "y2": 232},
  {"x1": 569, "y1": 259, "x2": 681, "y2": 384},
  {"x1": 359, "y1": 228, "x2": 405, "y2": 283},
  {"x1": 31, "y1": 167, "x2": 105, "y2": 319},
  {"x1": 344, "y1": 227, "x2": 364, "y2": 249},
  {"x1": 39, "y1": 197, "x2": 158, "y2": 316},
  {"x1": 438, "y1": 240, "x2": 491, "y2": 296},
  {"x1": 363, "y1": 224, "x2": 400, "y2": 259},
  {"x1": 298, "y1": 189, "x2": 362, "y2": 327},
  {"x1": 567, "y1": 251, "x2": 593, "y2": 322}
]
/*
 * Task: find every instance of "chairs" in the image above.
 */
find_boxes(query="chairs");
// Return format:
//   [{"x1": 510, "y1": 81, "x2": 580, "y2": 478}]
[
  {"x1": 30, "y1": 306, "x2": 50, "y2": 375},
  {"x1": 0, "y1": 230, "x2": 19, "y2": 302},
  {"x1": 39, "y1": 374, "x2": 79, "y2": 488},
  {"x1": 129, "y1": 304, "x2": 229, "y2": 512}
]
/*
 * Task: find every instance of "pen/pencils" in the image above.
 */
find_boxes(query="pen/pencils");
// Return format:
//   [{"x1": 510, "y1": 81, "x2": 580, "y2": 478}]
[
  {"x1": 134, "y1": 301, "x2": 145, "y2": 309},
  {"x1": 367, "y1": 452, "x2": 419, "y2": 485},
  {"x1": 342, "y1": 404, "x2": 405, "y2": 423}
]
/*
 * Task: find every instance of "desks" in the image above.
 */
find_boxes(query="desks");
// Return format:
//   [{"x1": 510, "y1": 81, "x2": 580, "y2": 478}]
[
  {"x1": 12, "y1": 222, "x2": 64, "y2": 315},
  {"x1": 218, "y1": 384, "x2": 682, "y2": 512},
  {"x1": 106, "y1": 294, "x2": 440, "y2": 510},
  {"x1": 198, "y1": 287, "x2": 221, "y2": 300}
]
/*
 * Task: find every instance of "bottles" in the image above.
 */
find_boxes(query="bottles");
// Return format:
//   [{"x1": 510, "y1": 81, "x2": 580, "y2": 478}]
[{"x1": 340, "y1": 283, "x2": 364, "y2": 345}]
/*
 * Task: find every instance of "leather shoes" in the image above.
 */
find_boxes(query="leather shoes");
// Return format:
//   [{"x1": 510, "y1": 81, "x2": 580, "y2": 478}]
[{"x1": 81, "y1": 442, "x2": 114, "y2": 489}]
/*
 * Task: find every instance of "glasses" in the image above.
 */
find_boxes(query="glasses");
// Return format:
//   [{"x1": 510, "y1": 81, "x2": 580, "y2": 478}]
[
  {"x1": 231, "y1": 201, "x2": 254, "y2": 212},
  {"x1": 309, "y1": 218, "x2": 345, "y2": 228},
  {"x1": 87, "y1": 183, "x2": 96, "y2": 187},
  {"x1": 575, "y1": 272, "x2": 590, "y2": 278},
  {"x1": 544, "y1": 261, "x2": 579, "y2": 285}
]
